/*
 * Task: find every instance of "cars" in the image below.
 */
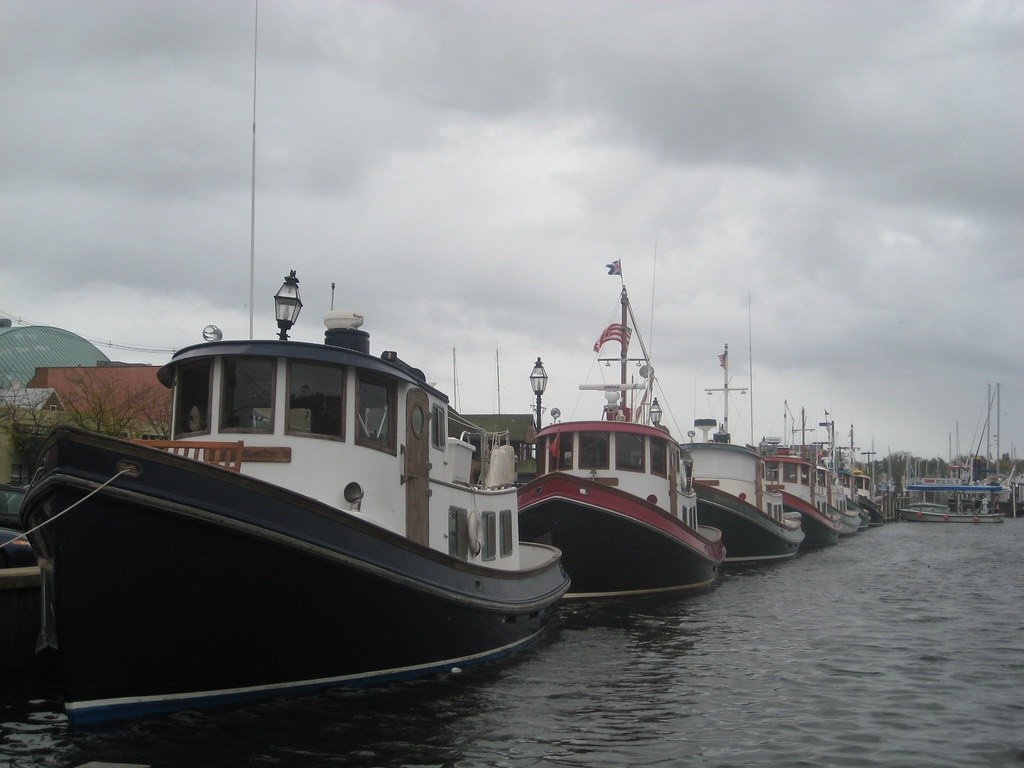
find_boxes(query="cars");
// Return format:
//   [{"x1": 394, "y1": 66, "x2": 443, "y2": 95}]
[{"x1": 0, "y1": 483, "x2": 40, "y2": 569}]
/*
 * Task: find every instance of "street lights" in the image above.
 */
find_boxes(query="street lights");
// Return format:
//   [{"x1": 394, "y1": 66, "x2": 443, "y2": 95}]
[{"x1": 527, "y1": 356, "x2": 548, "y2": 432}]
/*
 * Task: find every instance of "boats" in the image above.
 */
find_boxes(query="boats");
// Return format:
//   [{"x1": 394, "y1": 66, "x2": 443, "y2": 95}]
[
  {"x1": 18, "y1": 304, "x2": 572, "y2": 732},
  {"x1": 676, "y1": 374, "x2": 807, "y2": 569},
  {"x1": 504, "y1": 284, "x2": 727, "y2": 603},
  {"x1": 751, "y1": 381, "x2": 1023, "y2": 550}
]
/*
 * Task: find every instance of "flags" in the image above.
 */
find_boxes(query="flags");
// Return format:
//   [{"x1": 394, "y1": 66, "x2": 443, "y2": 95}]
[
  {"x1": 825, "y1": 410, "x2": 829, "y2": 415},
  {"x1": 718, "y1": 352, "x2": 727, "y2": 370},
  {"x1": 605, "y1": 258, "x2": 622, "y2": 276},
  {"x1": 547, "y1": 431, "x2": 560, "y2": 458}
]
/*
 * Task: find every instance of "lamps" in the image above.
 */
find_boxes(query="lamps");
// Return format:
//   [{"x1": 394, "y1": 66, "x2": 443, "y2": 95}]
[
  {"x1": 202, "y1": 324, "x2": 222, "y2": 342},
  {"x1": 688, "y1": 431, "x2": 695, "y2": 442},
  {"x1": 551, "y1": 408, "x2": 561, "y2": 424}
]
